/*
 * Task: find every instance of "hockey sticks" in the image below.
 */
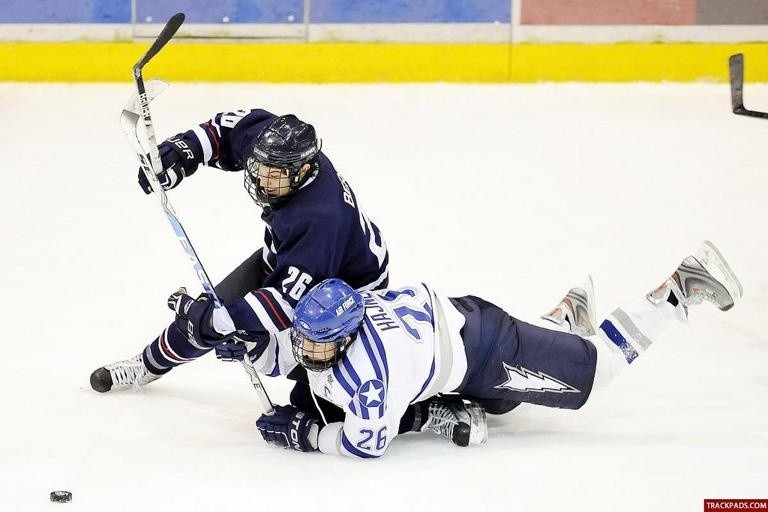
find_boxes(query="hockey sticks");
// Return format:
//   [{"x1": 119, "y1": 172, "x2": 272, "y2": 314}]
[
  {"x1": 119, "y1": 79, "x2": 276, "y2": 416},
  {"x1": 131, "y1": 11, "x2": 185, "y2": 176}
]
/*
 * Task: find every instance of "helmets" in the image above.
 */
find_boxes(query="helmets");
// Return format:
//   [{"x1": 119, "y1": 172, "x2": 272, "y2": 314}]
[
  {"x1": 289, "y1": 277, "x2": 366, "y2": 372},
  {"x1": 244, "y1": 114, "x2": 318, "y2": 215}
]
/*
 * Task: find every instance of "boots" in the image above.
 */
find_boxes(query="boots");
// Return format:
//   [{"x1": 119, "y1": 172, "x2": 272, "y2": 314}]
[
  {"x1": 647, "y1": 256, "x2": 734, "y2": 312},
  {"x1": 542, "y1": 287, "x2": 595, "y2": 337},
  {"x1": 420, "y1": 392, "x2": 472, "y2": 446},
  {"x1": 90, "y1": 353, "x2": 162, "y2": 393}
]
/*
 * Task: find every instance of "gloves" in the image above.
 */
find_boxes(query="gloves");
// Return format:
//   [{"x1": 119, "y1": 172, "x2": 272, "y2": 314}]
[
  {"x1": 167, "y1": 292, "x2": 237, "y2": 351},
  {"x1": 136, "y1": 132, "x2": 198, "y2": 195},
  {"x1": 256, "y1": 404, "x2": 321, "y2": 452},
  {"x1": 215, "y1": 332, "x2": 270, "y2": 362}
]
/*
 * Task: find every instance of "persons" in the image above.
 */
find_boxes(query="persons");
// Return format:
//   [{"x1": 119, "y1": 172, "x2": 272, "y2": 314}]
[
  {"x1": 215, "y1": 252, "x2": 735, "y2": 458},
  {"x1": 88, "y1": 106, "x2": 470, "y2": 448}
]
